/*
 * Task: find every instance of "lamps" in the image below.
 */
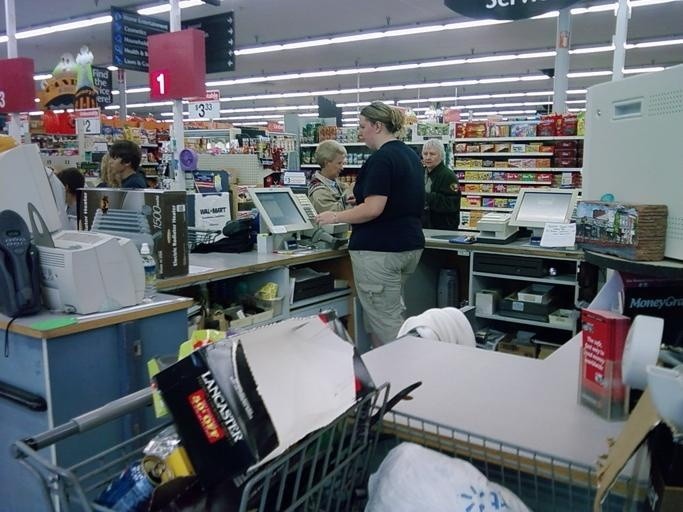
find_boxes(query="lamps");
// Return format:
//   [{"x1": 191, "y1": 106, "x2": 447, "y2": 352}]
[{"x1": 3, "y1": 1, "x2": 682, "y2": 125}]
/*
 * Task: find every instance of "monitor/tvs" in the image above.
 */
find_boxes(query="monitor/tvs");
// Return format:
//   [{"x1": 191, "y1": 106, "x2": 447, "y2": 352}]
[
  {"x1": 0, "y1": 141, "x2": 63, "y2": 235},
  {"x1": 247, "y1": 188, "x2": 313, "y2": 251},
  {"x1": 508, "y1": 188, "x2": 579, "y2": 237}
]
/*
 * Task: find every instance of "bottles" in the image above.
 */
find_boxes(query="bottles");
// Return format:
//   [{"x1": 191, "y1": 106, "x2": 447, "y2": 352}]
[
  {"x1": 139, "y1": 242, "x2": 158, "y2": 304},
  {"x1": 196, "y1": 283, "x2": 210, "y2": 317},
  {"x1": 42, "y1": 106, "x2": 73, "y2": 135},
  {"x1": 242, "y1": 138, "x2": 257, "y2": 154}
]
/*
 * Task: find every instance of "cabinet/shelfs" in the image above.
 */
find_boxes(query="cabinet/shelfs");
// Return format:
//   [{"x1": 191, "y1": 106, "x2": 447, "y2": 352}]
[
  {"x1": 453, "y1": 135, "x2": 586, "y2": 226},
  {"x1": 299, "y1": 139, "x2": 450, "y2": 211},
  {"x1": 16, "y1": 127, "x2": 87, "y2": 200},
  {"x1": 148, "y1": 241, "x2": 373, "y2": 362},
  {"x1": 194, "y1": 154, "x2": 266, "y2": 197},
  {"x1": 1, "y1": 295, "x2": 194, "y2": 507},
  {"x1": 422, "y1": 228, "x2": 586, "y2": 352},
  {"x1": 351, "y1": 331, "x2": 590, "y2": 508},
  {"x1": 91, "y1": 127, "x2": 186, "y2": 196}
]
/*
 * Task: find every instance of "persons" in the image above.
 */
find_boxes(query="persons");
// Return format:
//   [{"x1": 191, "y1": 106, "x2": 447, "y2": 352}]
[
  {"x1": 421, "y1": 138, "x2": 461, "y2": 230},
  {"x1": 306, "y1": 140, "x2": 356, "y2": 227},
  {"x1": 314, "y1": 102, "x2": 425, "y2": 345},
  {"x1": 56, "y1": 139, "x2": 150, "y2": 214}
]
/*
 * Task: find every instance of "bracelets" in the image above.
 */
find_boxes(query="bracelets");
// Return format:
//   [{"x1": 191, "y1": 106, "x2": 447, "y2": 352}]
[{"x1": 334, "y1": 212, "x2": 337, "y2": 223}]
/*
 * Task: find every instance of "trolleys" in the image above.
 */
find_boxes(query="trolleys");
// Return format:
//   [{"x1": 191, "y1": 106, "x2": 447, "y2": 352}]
[{"x1": 8, "y1": 307, "x2": 657, "y2": 512}]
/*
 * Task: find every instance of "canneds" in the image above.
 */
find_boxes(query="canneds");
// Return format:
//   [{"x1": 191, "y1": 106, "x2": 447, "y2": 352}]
[{"x1": 95, "y1": 454, "x2": 175, "y2": 512}]
